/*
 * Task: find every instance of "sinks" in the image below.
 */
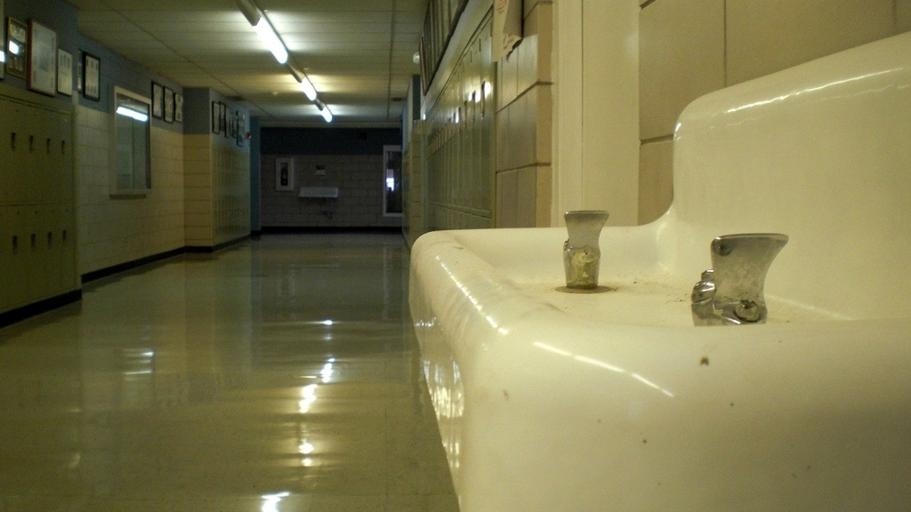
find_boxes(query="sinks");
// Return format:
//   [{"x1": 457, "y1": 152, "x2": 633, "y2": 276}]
[{"x1": 410, "y1": 226, "x2": 911, "y2": 512}]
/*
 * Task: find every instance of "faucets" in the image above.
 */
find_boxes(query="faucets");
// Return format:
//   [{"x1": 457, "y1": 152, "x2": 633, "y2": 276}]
[{"x1": 561, "y1": 210, "x2": 608, "y2": 287}]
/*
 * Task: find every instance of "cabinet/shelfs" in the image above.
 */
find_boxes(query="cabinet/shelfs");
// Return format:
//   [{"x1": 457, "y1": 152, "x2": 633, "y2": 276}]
[{"x1": 0, "y1": 82, "x2": 82, "y2": 327}]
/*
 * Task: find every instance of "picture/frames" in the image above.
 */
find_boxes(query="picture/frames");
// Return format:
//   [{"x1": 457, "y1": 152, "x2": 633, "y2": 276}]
[
  {"x1": 5, "y1": 15, "x2": 102, "y2": 102},
  {"x1": 150, "y1": 80, "x2": 184, "y2": 123},
  {"x1": 419, "y1": 0, "x2": 472, "y2": 96},
  {"x1": 212, "y1": 99, "x2": 246, "y2": 147}
]
